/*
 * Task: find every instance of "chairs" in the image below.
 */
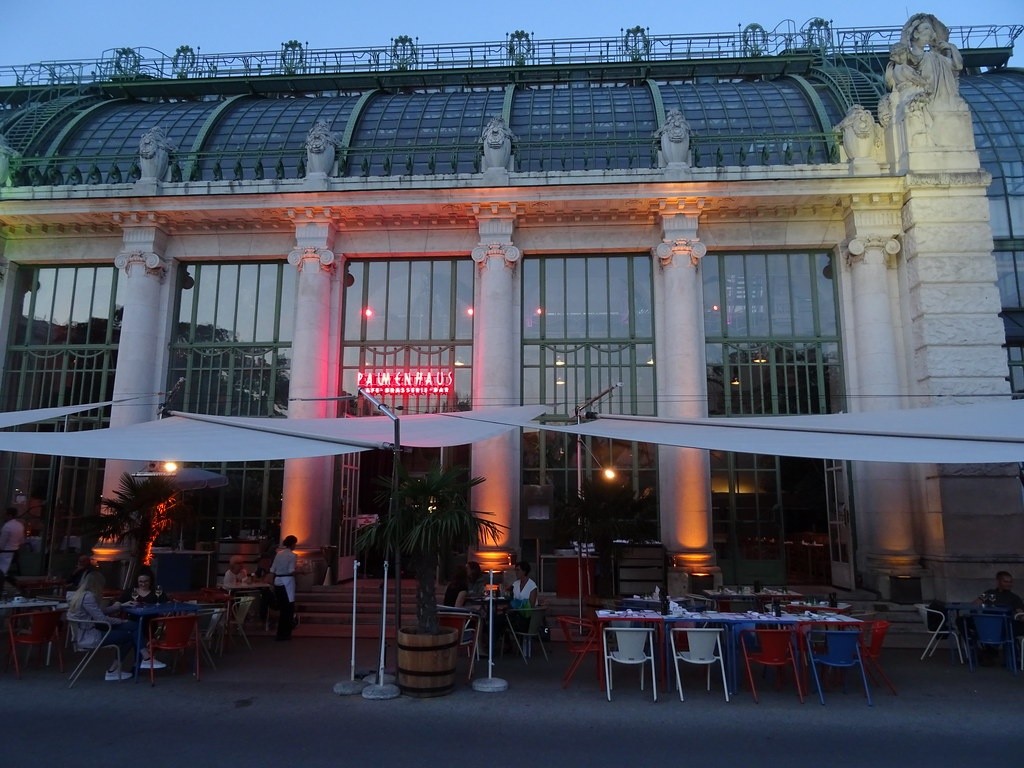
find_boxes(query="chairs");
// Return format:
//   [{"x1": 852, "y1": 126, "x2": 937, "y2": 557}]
[
  {"x1": 5, "y1": 595, "x2": 256, "y2": 689},
  {"x1": 16, "y1": 580, "x2": 65, "y2": 602},
  {"x1": 963, "y1": 613, "x2": 1018, "y2": 677},
  {"x1": 435, "y1": 604, "x2": 480, "y2": 658},
  {"x1": 1014, "y1": 613, "x2": 1024, "y2": 671},
  {"x1": 505, "y1": 598, "x2": 539, "y2": 659},
  {"x1": 436, "y1": 612, "x2": 481, "y2": 681},
  {"x1": 463, "y1": 586, "x2": 487, "y2": 615},
  {"x1": 560, "y1": 605, "x2": 899, "y2": 707},
  {"x1": 913, "y1": 604, "x2": 969, "y2": 665},
  {"x1": 505, "y1": 593, "x2": 556, "y2": 667}
]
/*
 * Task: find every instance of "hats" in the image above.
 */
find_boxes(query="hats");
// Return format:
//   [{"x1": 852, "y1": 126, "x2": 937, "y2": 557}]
[{"x1": 282, "y1": 535, "x2": 297, "y2": 547}]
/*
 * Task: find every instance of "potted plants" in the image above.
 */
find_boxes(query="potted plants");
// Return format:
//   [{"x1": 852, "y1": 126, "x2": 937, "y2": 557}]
[
  {"x1": 350, "y1": 451, "x2": 512, "y2": 699},
  {"x1": 543, "y1": 497, "x2": 583, "y2": 555}
]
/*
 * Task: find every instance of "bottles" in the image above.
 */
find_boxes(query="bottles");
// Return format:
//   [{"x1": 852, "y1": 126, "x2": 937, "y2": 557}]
[
  {"x1": 775, "y1": 603, "x2": 782, "y2": 617},
  {"x1": 754, "y1": 576, "x2": 760, "y2": 592},
  {"x1": 660, "y1": 593, "x2": 668, "y2": 615},
  {"x1": 828, "y1": 592, "x2": 838, "y2": 607}
]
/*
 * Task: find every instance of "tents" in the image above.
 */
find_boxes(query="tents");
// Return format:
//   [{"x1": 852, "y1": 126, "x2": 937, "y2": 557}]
[
  {"x1": 535, "y1": 397, "x2": 1024, "y2": 639},
  {"x1": 1, "y1": 404, "x2": 545, "y2": 701},
  {"x1": 1, "y1": 393, "x2": 167, "y2": 426}
]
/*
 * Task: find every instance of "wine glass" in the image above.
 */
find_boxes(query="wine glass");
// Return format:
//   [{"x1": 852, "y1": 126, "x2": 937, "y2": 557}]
[
  {"x1": 131, "y1": 587, "x2": 140, "y2": 605},
  {"x1": 498, "y1": 584, "x2": 506, "y2": 599},
  {"x1": 154, "y1": 584, "x2": 163, "y2": 606},
  {"x1": 981, "y1": 593, "x2": 987, "y2": 609},
  {"x1": 989, "y1": 594, "x2": 995, "y2": 608}
]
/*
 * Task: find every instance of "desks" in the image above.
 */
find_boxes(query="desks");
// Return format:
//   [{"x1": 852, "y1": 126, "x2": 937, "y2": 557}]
[{"x1": 152, "y1": 546, "x2": 214, "y2": 589}]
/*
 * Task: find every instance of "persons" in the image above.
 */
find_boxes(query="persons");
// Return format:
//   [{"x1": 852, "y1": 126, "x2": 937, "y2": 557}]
[
  {"x1": 955, "y1": 571, "x2": 1024, "y2": 639},
  {"x1": 0, "y1": 509, "x2": 24, "y2": 596},
  {"x1": 223, "y1": 554, "x2": 244, "y2": 591},
  {"x1": 67, "y1": 572, "x2": 168, "y2": 681},
  {"x1": 506, "y1": 561, "x2": 537, "y2": 632},
  {"x1": 72, "y1": 555, "x2": 92, "y2": 592},
  {"x1": 440, "y1": 561, "x2": 490, "y2": 658},
  {"x1": 270, "y1": 535, "x2": 295, "y2": 631},
  {"x1": 884, "y1": 12, "x2": 968, "y2": 111}
]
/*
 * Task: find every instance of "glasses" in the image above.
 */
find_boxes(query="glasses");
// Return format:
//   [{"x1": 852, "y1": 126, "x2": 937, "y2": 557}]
[{"x1": 137, "y1": 579, "x2": 149, "y2": 584}]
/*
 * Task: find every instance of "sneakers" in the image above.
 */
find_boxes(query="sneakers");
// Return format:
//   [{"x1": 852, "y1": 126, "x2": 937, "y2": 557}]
[
  {"x1": 104, "y1": 669, "x2": 132, "y2": 681},
  {"x1": 140, "y1": 657, "x2": 166, "y2": 668}
]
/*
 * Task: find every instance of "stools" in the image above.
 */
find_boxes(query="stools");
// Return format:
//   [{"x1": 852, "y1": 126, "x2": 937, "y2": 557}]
[{"x1": 761, "y1": 537, "x2": 823, "y2": 577}]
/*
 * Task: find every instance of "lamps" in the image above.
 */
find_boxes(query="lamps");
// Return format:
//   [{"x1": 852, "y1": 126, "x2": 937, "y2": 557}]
[
  {"x1": 688, "y1": 571, "x2": 714, "y2": 595},
  {"x1": 483, "y1": 570, "x2": 504, "y2": 585},
  {"x1": 889, "y1": 574, "x2": 923, "y2": 605},
  {"x1": 97, "y1": 560, "x2": 122, "y2": 588}
]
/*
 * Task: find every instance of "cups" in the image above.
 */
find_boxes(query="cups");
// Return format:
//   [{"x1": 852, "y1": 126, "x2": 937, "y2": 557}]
[
  {"x1": 781, "y1": 587, "x2": 788, "y2": 594},
  {"x1": 737, "y1": 586, "x2": 744, "y2": 594},
  {"x1": 14, "y1": 596, "x2": 25, "y2": 603}
]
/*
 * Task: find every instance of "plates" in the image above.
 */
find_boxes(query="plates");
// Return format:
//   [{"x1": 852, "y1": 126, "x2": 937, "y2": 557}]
[{"x1": 12, "y1": 601, "x2": 26, "y2": 604}]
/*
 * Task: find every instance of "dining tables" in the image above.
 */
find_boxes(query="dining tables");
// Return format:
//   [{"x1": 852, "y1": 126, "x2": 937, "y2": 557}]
[
  {"x1": 0, "y1": 596, "x2": 69, "y2": 665},
  {"x1": 944, "y1": 602, "x2": 1024, "y2": 667},
  {"x1": 469, "y1": 597, "x2": 507, "y2": 657},
  {"x1": 121, "y1": 602, "x2": 198, "y2": 685},
  {"x1": 224, "y1": 582, "x2": 270, "y2": 631},
  {"x1": 595, "y1": 588, "x2": 852, "y2": 694}
]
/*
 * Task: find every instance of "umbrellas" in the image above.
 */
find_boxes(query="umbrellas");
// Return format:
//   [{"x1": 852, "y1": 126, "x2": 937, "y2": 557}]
[{"x1": 166, "y1": 468, "x2": 228, "y2": 552}]
